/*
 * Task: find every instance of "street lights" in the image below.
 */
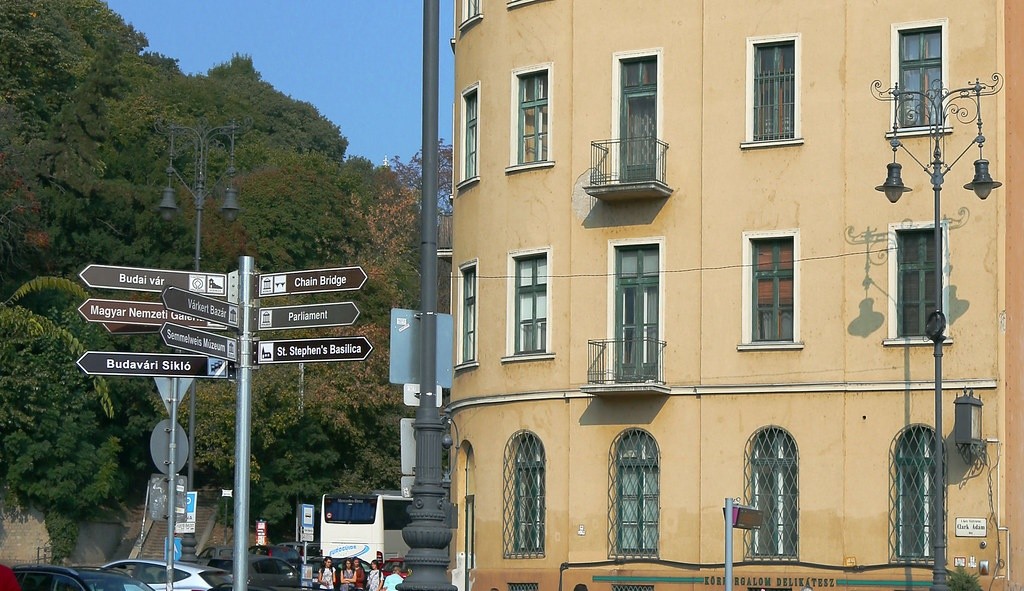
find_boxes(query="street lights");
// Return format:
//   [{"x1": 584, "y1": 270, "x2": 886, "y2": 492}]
[
  {"x1": 870, "y1": 72, "x2": 1007, "y2": 590},
  {"x1": 158, "y1": 121, "x2": 242, "y2": 564}
]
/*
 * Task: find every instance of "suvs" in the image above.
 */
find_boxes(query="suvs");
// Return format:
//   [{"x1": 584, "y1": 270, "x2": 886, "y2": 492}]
[
  {"x1": 9, "y1": 562, "x2": 157, "y2": 591},
  {"x1": 93, "y1": 558, "x2": 235, "y2": 591}
]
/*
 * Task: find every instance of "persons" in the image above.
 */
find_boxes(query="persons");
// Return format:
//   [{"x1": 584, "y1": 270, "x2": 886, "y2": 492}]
[
  {"x1": 383, "y1": 567, "x2": 404, "y2": 591},
  {"x1": 366, "y1": 560, "x2": 384, "y2": 591},
  {"x1": 295, "y1": 547, "x2": 304, "y2": 572},
  {"x1": 352, "y1": 557, "x2": 365, "y2": 591},
  {"x1": 126, "y1": 569, "x2": 133, "y2": 576},
  {"x1": 406, "y1": 568, "x2": 413, "y2": 578},
  {"x1": 318, "y1": 556, "x2": 336, "y2": 591},
  {"x1": 340, "y1": 558, "x2": 357, "y2": 591},
  {"x1": 256, "y1": 546, "x2": 265, "y2": 554}
]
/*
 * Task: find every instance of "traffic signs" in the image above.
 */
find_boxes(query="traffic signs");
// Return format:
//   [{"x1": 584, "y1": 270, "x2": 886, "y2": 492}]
[
  {"x1": 258, "y1": 301, "x2": 361, "y2": 331},
  {"x1": 76, "y1": 350, "x2": 228, "y2": 379},
  {"x1": 258, "y1": 265, "x2": 367, "y2": 298},
  {"x1": 77, "y1": 298, "x2": 228, "y2": 331},
  {"x1": 161, "y1": 285, "x2": 239, "y2": 328},
  {"x1": 257, "y1": 336, "x2": 373, "y2": 365},
  {"x1": 158, "y1": 321, "x2": 237, "y2": 362},
  {"x1": 78, "y1": 264, "x2": 227, "y2": 297}
]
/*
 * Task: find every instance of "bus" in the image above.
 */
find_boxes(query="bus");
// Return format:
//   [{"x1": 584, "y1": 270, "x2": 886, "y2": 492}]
[{"x1": 318, "y1": 492, "x2": 410, "y2": 570}]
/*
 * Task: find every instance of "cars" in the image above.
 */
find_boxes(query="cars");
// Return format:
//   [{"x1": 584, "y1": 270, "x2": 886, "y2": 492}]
[{"x1": 197, "y1": 540, "x2": 403, "y2": 591}]
